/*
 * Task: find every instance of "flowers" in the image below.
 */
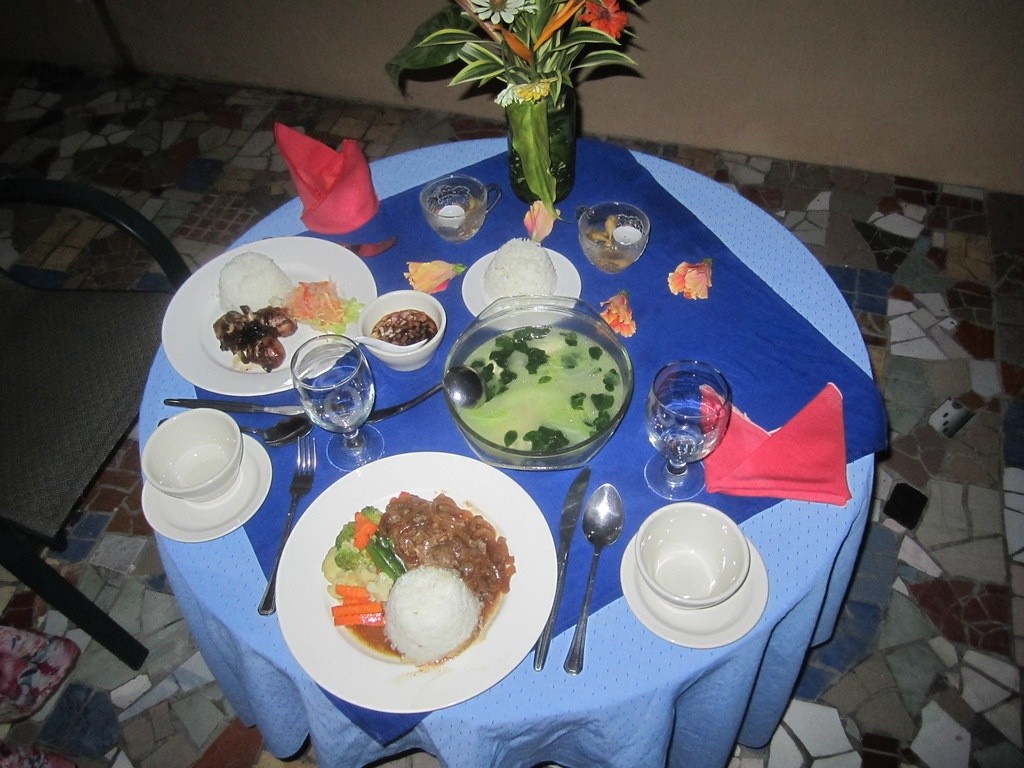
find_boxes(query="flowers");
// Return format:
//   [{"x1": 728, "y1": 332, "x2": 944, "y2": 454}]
[
  {"x1": 523, "y1": 199, "x2": 562, "y2": 242},
  {"x1": 385, "y1": 0, "x2": 650, "y2": 224},
  {"x1": 402, "y1": 261, "x2": 467, "y2": 294},
  {"x1": 667, "y1": 257, "x2": 714, "y2": 300},
  {"x1": 600, "y1": 290, "x2": 636, "y2": 339}
]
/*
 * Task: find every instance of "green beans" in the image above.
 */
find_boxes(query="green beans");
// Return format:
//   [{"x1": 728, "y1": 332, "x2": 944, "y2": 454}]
[{"x1": 367, "y1": 534, "x2": 410, "y2": 580}]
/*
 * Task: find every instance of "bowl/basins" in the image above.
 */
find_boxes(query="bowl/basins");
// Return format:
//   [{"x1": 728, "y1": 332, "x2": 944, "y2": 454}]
[
  {"x1": 358, "y1": 290, "x2": 447, "y2": 371},
  {"x1": 443, "y1": 293, "x2": 635, "y2": 471},
  {"x1": 141, "y1": 408, "x2": 244, "y2": 503},
  {"x1": 635, "y1": 502, "x2": 751, "y2": 610}
]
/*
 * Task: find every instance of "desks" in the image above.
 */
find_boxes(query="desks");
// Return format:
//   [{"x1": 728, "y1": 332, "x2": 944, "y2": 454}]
[{"x1": 138, "y1": 137, "x2": 888, "y2": 768}]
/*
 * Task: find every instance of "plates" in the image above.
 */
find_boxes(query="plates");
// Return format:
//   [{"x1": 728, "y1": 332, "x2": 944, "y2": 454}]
[
  {"x1": 619, "y1": 533, "x2": 769, "y2": 649},
  {"x1": 460, "y1": 249, "x2": 582, "y2": 329},
  {"x1": 142, "y1": 433, "x2": 273, "y2": 544},
  {"x1": 274, "y1": 448, "x2": 557, "y2": 715},
  {"x1": 161, "y1": 234, "x2": 377, "y2": 397}
]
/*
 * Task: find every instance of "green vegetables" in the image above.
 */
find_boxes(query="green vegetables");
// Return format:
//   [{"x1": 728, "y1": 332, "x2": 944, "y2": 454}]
[{"x1": 469, "y1": 324, "x2": 620, "y2": 454}]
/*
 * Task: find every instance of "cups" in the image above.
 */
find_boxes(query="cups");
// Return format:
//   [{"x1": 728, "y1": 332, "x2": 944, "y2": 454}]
[
  {"x1": 419, "y1": 174, "x2": 503, "y2": 242},
  {"x1": 576, "y1": 201, "x2": 651, "y2": 274}
]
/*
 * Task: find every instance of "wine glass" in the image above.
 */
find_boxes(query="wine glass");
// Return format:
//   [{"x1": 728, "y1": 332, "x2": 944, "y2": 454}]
[
  {"x1": 643, "y1": 360, "x2": 732, "y2": 503},
  {"x1": 290, "y1": 333, "x2": 387, "y2": 473}
]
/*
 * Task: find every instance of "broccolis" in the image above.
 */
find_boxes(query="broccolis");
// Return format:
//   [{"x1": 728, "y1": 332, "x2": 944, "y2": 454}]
[{"x1": 334, "y1": 505, "x2": 382, "y2": 568}]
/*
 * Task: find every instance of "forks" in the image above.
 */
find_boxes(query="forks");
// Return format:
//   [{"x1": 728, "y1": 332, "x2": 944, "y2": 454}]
[{"x1": 257, "y1": 436, "x2": 318, "y2": 617}]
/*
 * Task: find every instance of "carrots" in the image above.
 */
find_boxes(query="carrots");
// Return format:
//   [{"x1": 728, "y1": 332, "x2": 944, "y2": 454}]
[
  {"x1": 330, "y1": 583, "x2": 385, "y2": 626},
  {"x1": 354, "y1": 512, "x2": 378, "y2": 549}
]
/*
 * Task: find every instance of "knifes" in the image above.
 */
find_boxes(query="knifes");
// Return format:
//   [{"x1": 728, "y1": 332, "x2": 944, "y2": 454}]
[
  {"x1": 533, "y1": 465, "x2": 592, "y2": 671},
  {"x1": 163, "y1": 399, "x2": 305, "y2": 417}
]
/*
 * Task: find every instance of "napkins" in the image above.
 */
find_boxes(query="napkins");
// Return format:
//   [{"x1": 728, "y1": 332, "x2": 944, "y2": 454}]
[
  {"x1": 274, "y1": 120, "x2": 380, "y2": 236},
  {"x1": 698, "y1": 382, "x2": 850, "y2": 506}
]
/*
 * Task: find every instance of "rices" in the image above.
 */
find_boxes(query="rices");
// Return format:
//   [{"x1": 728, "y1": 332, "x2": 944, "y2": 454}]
[
  {"x1": 484, "y1": 236, "x2": 558, "y2": 305},
  {"x1": 218, "y1": 251, "x2": 292, "y2": 312},
  {"x1": 382, "y1": 564, "x2": 481, "y2": 665}
]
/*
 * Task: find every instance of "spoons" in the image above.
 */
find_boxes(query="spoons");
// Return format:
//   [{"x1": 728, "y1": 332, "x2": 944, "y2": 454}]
[
  {"x1": 156, "y1": 417, "x2": 314, "y2": 448},
  {"x1": 564, "y1": 482, "x2": 624, "y2": 676},
  {"x1": 353, "y1": 335, "x2": 428, "y2": 353}
]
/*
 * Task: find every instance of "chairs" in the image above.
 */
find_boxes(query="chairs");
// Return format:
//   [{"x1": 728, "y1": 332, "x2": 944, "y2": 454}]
[{"x1": 0, "y1": 175, "x2": 194, "y2": 672}]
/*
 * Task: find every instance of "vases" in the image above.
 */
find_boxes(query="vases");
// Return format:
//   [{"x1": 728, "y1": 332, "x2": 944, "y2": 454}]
[{"x1": 504, "y1": 82, "x2": 575, "y2": 206}]
